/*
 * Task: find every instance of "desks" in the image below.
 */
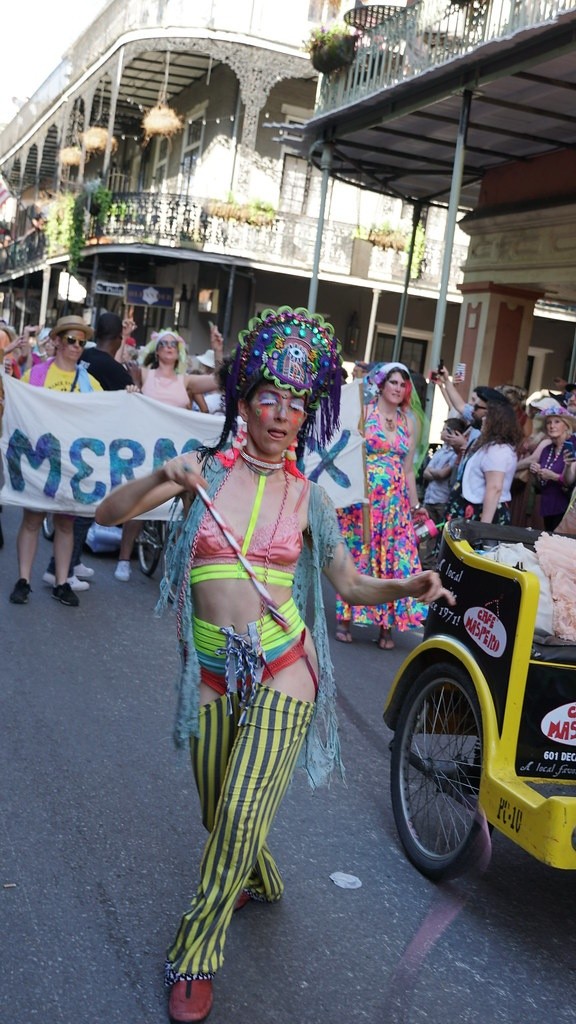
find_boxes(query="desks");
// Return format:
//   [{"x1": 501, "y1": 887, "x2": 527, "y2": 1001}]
[{"x1": 344, "y1": 5, "x2": 417, "y2": 80}]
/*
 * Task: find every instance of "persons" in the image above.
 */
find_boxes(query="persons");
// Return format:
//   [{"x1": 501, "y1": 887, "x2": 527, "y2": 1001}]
[
  {"x1": 0, "y1": 312, "x2": 140, "y2": 606},
  {"x1": 114, "y1": 310, "x2": 224, "y2": 580},
  {"x1": 336, "y1": 362, "x2": 428, "y2": 651},
  {"x1": 96, "y1": 306, "x2": 457, "y2": 1023},
  {"x1": 341, "y1": 359, "x2": 370, "y2": 384},
  {"x1": 0, "y1": 203, "x2": 48, "y2": 272},
  {"x1": 423, "y1": 365, "x2": 576, "y2": 570}
]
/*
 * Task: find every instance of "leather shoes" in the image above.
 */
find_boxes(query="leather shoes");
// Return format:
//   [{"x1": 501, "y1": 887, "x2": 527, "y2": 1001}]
[
  {"x1": 167, "y1": 975, "x2": 214, "y2": 1024},
  {"x1": 231, "y1": 888, "x2": 252, "y2": 913}
]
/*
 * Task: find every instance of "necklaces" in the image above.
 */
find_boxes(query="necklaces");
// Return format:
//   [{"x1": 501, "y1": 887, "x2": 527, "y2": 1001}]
[
  {"x1": 240, "y1": 447, "x2": 284, "y2": 469},
  {"x1": 380, "y1": 407, "x2": 398, "y2": 432},
  {"x1": 541, "y1": 444, "x2": 566, "y2": 485}
]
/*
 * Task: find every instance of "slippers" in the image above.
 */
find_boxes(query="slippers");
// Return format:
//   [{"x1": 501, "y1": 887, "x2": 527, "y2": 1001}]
[
  {"x1": 334, "y1": 624, "x2": 353, "y2": 644},
  {"x1": 377, "y1": 630, "x2": 394, "y2": 650}
]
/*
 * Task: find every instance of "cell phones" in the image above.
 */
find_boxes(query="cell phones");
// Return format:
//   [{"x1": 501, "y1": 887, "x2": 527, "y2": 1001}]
[
  {"x1": 445, "y1": 427, "x2": 452, "y2": 435},
  {"x1": 439, "y1": 359, "x2": 444, "y2": 375},
  {"x1": 429, "y1": 370, "x2": 441, "y2": 384}
]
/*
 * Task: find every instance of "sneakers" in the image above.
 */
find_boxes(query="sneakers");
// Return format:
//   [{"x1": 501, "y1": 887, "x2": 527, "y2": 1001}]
[
  {"x1": 9, "y1": 578, "x2": 33, "y2": 604},
  {"x1": 72, "y1": 564, "x2": 95, "y2": 576},
  {"x1": 51, "y1": 582, "x2": 79, "y2": 606},
  {"x1": 114, "y1": 559, "x2": 130, "y2": 581},
  {"x1": 43, "y1": 571, "x2": 56, "y2": 587},
  {"x1": 66, "y1": 576, "x2": 90, "y2": 590}
]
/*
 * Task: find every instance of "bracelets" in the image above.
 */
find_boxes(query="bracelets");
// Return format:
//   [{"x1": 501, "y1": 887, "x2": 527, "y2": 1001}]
[{"x1": 411, "y1": 502, "x2": 422, "y2": 511}]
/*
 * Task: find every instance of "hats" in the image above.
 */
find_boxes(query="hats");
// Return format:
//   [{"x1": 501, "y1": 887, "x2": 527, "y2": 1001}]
[
  {"x1": 566, "y1": 382, "x2": 576, "y2": 393},
  {"x1": 529, "y1": 397, "x2": 560, "y2": 416},
  {"x1": 476, "y1": 386, "x2": 508, "y2": 404},
  {"x1": 49, "y1": 316, "x2": 94, "y2": 340},
  {"x1": 541, "y1": 407, "x2": 573, "y2": 423},
  {"x1": 371, "y1": 363, "x2": 411, "y2": 385}
]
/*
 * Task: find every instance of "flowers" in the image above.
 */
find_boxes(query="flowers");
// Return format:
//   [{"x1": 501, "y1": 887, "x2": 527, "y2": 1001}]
[{"x1": 303, "y1": 26, "x2": 351, "y2": 55}]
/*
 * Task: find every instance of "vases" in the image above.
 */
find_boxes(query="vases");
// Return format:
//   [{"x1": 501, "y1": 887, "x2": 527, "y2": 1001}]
[{"x1": 311, "y1": 36, "x2": 356, "y2": 74}]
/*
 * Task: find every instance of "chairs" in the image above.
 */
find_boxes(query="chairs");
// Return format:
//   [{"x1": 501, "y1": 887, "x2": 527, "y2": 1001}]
[{"x1": 422, "y1": 519, "x2": 576, "y2": 780}]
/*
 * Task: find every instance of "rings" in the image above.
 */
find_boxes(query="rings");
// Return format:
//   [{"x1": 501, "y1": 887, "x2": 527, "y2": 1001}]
[{"x1": 180, "y1": 463, "x2": 190, "y2": 472}]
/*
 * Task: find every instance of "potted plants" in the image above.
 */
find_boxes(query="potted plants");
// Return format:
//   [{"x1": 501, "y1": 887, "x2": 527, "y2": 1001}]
[{"x1": 44, "y1": 185, "x2": 112, "y2": 279}]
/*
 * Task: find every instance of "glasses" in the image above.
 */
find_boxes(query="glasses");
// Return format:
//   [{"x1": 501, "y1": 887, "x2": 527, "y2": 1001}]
[
  {"x1": 158, "y1": 340, "x2": 178, "y2": 349},
  {"x1": 474, "y1": 404, "x2": 487, "y2": 411},
  {"x1": 59, "y1": 335, "x2": 86, "y2": 347}
]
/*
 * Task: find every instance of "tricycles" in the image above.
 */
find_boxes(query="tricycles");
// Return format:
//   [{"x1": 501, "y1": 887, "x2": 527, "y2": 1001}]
[
  {"x1": 43, "y1": 512, "x2": 166, "y2": 576},
  {"x1": 382, "y1": 519, "x2": 576, "y2": 879}
]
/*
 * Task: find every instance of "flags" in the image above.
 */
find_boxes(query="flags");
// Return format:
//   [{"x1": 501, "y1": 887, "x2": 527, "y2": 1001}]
[{"x1": 0, "y1": 172, "x2": 11, "y2": 209}]
